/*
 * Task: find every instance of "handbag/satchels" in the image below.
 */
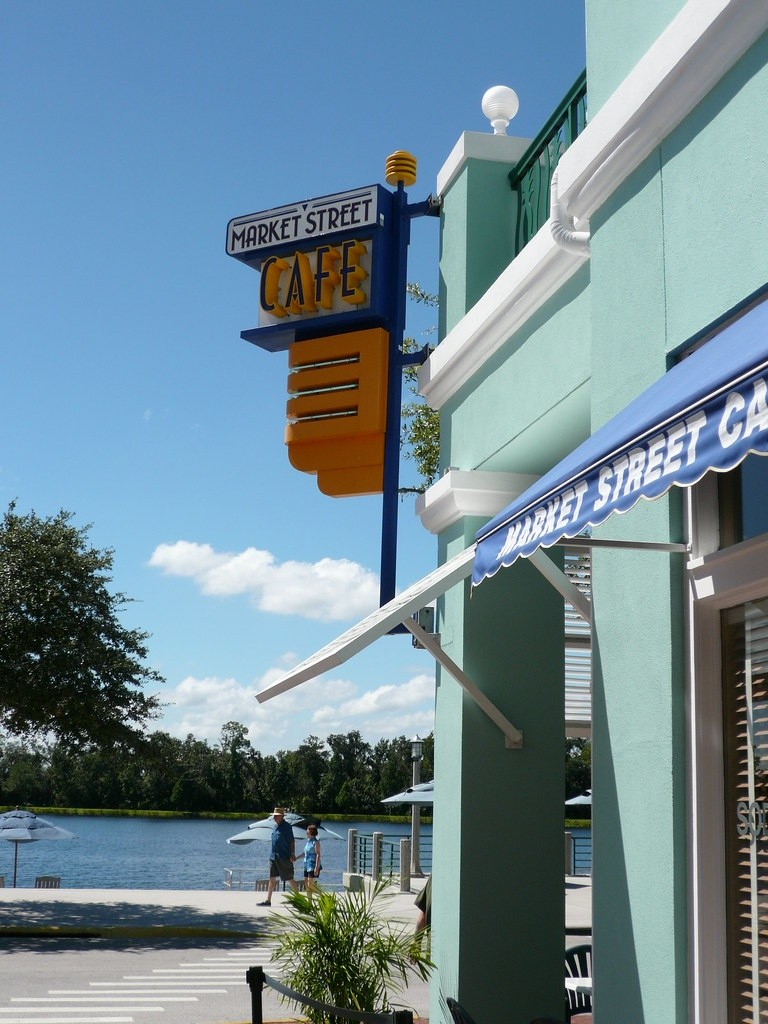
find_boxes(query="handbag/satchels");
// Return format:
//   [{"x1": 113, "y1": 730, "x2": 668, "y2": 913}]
[{"x1": 313, "y1": 862, "x2": 322, "y2": 871}]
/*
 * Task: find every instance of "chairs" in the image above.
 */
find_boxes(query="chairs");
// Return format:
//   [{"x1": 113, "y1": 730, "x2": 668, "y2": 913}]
[
  {"x1": 0, "y1": 876, "x2": 60, "y2": 889},
  {"x1": 255, "y1": 878, "x2": 279, "y2": 892},
  {"x1": 447, "y1": 944, "x2": 593, "y2": 1024},
  {"x1": 295, "y1": 881, "x2": 317, "y2": 892}
]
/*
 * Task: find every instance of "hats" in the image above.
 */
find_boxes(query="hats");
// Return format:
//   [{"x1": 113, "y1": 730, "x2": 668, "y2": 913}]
[{"x1": 269, "y1": 807, "x2": 287, "y2": 816}]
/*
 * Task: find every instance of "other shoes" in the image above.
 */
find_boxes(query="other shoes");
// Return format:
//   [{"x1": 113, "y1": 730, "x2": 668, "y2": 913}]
[{"x1": 256, "y1": 900, "x2": 271, "y2": 906}]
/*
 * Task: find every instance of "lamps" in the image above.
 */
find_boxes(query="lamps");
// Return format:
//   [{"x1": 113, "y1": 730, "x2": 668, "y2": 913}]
[{"x1": 481, "y1": 85, "x2": 520, "y2": 136}]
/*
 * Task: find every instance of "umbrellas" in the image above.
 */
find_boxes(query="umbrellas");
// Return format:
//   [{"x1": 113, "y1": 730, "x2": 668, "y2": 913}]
[
  {"x1": 0, "y1": 806, "x2": 79, "y2": 888},
  {"x1": 226, "y1": 808, "x2": 346, "y2": 891},
  {"x1": 380, "y1": 779, "x2": 434, "y2": 806},
  {"x1": 565, "y1": 789, "x2": 592, "y2": 805}
]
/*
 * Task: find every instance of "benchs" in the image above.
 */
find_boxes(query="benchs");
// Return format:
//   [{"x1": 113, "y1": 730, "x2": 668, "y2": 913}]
[{"x1": 23, "y1": 802, "x2": 32, "y2": 807}]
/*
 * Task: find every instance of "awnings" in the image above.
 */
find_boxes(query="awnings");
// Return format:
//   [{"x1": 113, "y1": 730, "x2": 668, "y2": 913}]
[{"x1": 471, "y1": 300, "x2": 768, "y2": 598}]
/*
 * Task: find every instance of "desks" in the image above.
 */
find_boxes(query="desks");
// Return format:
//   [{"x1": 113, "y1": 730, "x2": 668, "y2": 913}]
[{"x1": 563, "y1": 977, "x2": 593, "y2": 996}]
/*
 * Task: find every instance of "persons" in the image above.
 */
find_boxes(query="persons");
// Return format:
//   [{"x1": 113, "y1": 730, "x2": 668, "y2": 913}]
[
  {"x1": 290, "y1": 825, "x2": 325, "y2": 906},
  {"x1": 255, "y1": 808, "x2": 299, "y2": 906},
  {"x1": 408, "y1": 872, "x2": 431, "y2": 965}
]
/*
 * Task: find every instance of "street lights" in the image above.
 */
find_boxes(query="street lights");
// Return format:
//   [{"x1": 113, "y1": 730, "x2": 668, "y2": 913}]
[{"x1": 409, "y1": 733, "x2": 426, "y2": 878}]
